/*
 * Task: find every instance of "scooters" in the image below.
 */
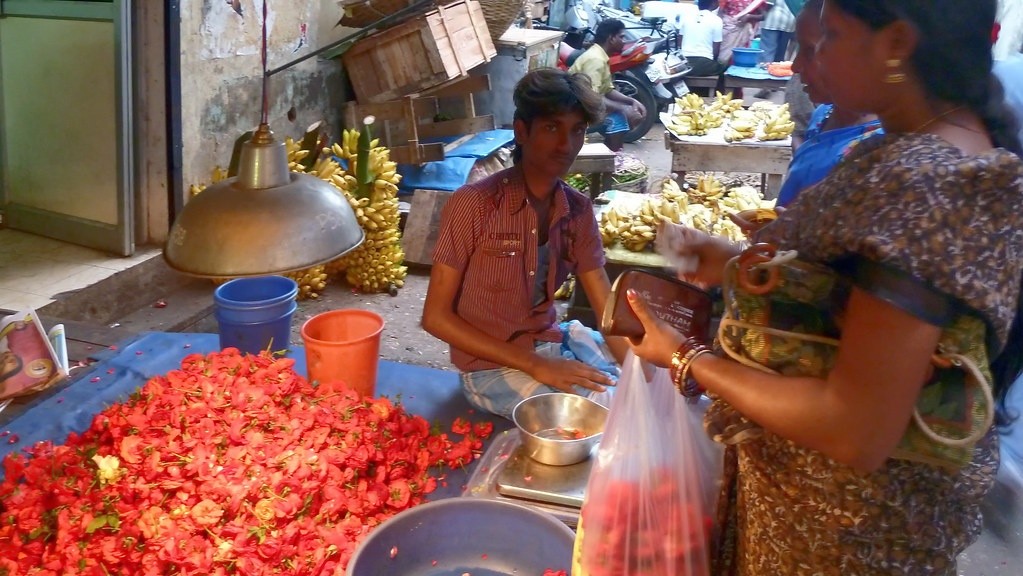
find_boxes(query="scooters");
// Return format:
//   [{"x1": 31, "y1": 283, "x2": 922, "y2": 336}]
[{"x1": 520, "y1": 0, "x2": 694, "y2": 151}]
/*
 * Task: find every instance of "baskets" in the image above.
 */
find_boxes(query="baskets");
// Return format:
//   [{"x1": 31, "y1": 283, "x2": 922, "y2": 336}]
[{"x1": 478, "y1": 1, "x2": 526, "y2": 41}]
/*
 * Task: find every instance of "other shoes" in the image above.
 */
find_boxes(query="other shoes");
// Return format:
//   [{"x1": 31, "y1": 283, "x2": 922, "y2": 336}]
[{"x1": 753, "y1": 88, "x2": 771, "y2": 98}]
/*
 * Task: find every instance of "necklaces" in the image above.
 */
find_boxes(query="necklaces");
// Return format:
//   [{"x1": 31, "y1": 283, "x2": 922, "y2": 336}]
[{"x1": 910, "y1": 101, "x2": 969, "y2": 134}]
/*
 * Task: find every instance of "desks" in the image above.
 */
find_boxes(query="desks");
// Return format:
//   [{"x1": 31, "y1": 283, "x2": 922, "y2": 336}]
[
  {"x1": 723, "y1": 65, "x2": 793, "y2": 101},
  {"x1": 664, "y1": 97, "x2": 794, "y2": 201}
]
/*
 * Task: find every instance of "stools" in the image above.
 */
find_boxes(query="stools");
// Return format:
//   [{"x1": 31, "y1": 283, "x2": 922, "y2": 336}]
[
  {"x1": 682, "y1": 74, "x2": 721, "y2": 97},
  {"x1": 566, "y1": 142, "x2": 616, "y2": 199}
]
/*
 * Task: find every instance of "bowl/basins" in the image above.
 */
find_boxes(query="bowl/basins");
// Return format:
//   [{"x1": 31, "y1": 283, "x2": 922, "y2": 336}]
[
  {"x1": 730, "y1": 47, "x2": 764, "y2": 66},
  {"x1": 343, "y1": 496, "x2": 578, "y2": 576},
  {"x1": 512, "y1": 392, "x2": 611, "y2": 467}
]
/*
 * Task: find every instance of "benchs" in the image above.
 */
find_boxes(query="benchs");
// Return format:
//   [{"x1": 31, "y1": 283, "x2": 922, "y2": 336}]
[{"x1": 345, "y1": 72, "x2": 496, "y2": 169}]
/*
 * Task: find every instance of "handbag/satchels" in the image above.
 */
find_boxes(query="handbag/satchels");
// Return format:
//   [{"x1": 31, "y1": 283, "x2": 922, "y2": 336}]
[
  {"x1": 718, "y1": 242, "x2": 993, "y2": 476},
  {"x1": 572, "y1": 347, "x2": 724, "y2": 576}
]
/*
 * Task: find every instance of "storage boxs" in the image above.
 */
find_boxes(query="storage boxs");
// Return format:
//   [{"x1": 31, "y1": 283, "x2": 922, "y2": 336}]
[{"x1": 342, "y1": 1, "x2": 498, "y2": 106}]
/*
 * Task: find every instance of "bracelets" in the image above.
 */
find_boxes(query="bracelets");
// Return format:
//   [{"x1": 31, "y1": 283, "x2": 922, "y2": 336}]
[{"x1": 669, "y1": 335, "x2": 715, "y2": 398}]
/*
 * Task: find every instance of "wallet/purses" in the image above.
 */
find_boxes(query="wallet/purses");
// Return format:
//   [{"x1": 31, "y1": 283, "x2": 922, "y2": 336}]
[{"x1": 598, "y1": 270, "x2": 713, "y2": 352}]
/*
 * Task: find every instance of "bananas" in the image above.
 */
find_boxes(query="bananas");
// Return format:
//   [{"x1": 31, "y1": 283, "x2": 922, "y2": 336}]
[
  {"x1": 554, "y1": 275, "x2": 577, "y2": 299},
  {"x1": 596, "y1": 175, "x2": 765, "y2": 253},
  {"x1": 188, "y1": 126, "x2": 409, "y2": 300},
  {"x1": 667, "y1": 91, "x2": 794, "y2": 142}
]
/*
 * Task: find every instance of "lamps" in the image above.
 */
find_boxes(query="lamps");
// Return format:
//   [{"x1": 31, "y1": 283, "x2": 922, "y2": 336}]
[{"x1": 161, "y1": 1, "x2": 365, "y2": 279}]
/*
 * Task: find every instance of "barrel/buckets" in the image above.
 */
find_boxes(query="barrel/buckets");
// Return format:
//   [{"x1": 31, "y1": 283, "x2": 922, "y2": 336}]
[
  {"x1": 300, "y1": 308, "x2": 385, "y2": 402},
  {"x1": 211, "y1": 275, "x2": 298, "y2": 360}
]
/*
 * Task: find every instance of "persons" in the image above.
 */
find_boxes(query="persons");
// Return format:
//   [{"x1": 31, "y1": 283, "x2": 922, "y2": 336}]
[
  {"x1": 421, "y1": 68, "x2": 658, "y2": 425},
  {"x1": 623, "y1": 0, "x2": 1023, "y2": 576},
  {"x1": 679, "y1": 0, "x2": 726, "y2": 98},
  {"x1": 726, "y1": 1, "x2": 886, "y2": 244},
  {"x1": 731, "y1": 0, "x2": 797, "y2": 100},
  {"x1": 568, "y1": 18, "x2": 647, "y2": 154},
  {"x1": 710, "y1": 1, "x2": 768, "y2": 96}
]
